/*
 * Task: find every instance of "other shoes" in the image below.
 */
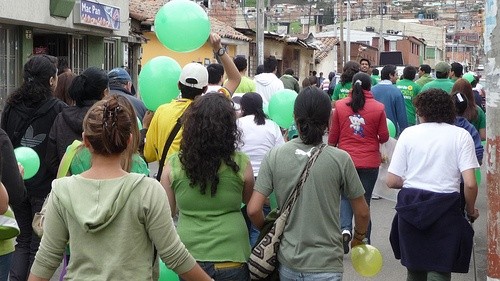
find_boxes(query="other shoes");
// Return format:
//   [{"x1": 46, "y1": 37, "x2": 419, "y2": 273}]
[
  {"x1": 342, "y1": 229, "x2": 352, "y2": 254},
  {"x1": 372, "y1": 194, "x2": 382, "y2": 200}
]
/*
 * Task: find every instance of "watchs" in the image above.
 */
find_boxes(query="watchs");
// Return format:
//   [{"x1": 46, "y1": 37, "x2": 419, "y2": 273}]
[{"x1": 213, "y1": 47, "x2": 226, "y2": 56}]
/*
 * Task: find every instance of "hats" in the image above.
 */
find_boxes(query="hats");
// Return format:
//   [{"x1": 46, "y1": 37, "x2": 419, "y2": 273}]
[
  {"x1": 179, "y1": 62, "x2": 209, "y2": 89},
  {"x1": 463, "y1": 74, "x2": 477, "y2": 86},
  {"x1": 312, "y1": 71, "x2": 316, "y2": 74},
  {"x1": 108, "y1": 67, "x2": 136, "y2": 96},
  {"x1": 320, "y1": 72, "x2": 323, "y2": 75},
  {"x1": 256, "y1": 66, "x2": 264, "y2": 73},
  {"x1": 285, "y1": 69, "x2": 295, "y2": 75},
  {"x1": 436, "y1": 62, "x2": 452, "y2": 75},
  {"x1": 54, "y1": 71, "x2": 78, "y2": 100},
  {"x1": 264, "y1": 55, "x2": 277, "y2": 67}
]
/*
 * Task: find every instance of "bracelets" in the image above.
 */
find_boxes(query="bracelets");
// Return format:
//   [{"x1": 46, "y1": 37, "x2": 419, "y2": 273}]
[{"x1": 468, "y1": 210, "x2": 476, "y2": 215}]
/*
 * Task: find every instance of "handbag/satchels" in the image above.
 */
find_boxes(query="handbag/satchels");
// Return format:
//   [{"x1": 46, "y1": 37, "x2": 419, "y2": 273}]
[
  {"x1": 0, "y1": 204, "x2": 21, "y2": 241},
  {"x1": 244, "y1": 144, "x2": 327, "y2": 281}
]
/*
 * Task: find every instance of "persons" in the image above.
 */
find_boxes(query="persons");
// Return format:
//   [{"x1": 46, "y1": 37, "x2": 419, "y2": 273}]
[
  {"x1": 450, "y1": 78, "x2": 486, "y2": 222},
  {"x1": 248, "y1": 85, "x2": 371, "y2": 281},
  {"x1": 0, "y1": 33, "x2": 302, "y2": 281},
  {"x1": 26, "y1": 100, "x2": 215, "y2": 281},
  {"x1": 302, "y1": 58, "x2": 485, "y2": 255},
  {"x1": 387, "y1": 87, "x2": 481, "y2": 281},
  {"x1": 159, "y1": 90, "x2": 265, "y2": 281}
]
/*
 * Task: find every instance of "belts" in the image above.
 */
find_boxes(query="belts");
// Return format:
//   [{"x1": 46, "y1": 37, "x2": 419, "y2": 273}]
[{"x1": 214, "y1": 262, "x2": 241, "y2": 268}]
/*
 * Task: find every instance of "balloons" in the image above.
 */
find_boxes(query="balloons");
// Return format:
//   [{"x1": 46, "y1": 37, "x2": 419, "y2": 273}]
[
  {"x1": 463, "y1": 73, "x2": 474, "y2": 83},
  {"x1": 154, "y1": 0, "x2": 211, "y2": 52},
  {"x1": 268, "y1": 89, "x2": 300, "y2": 129},
  {"x1": 385, "y1": 117, "x2": 397, "y2": 138},
  {"x1": 350, "y1": 242, "x2": 382, "y2": 278},
  {"x1": 13, "y1": 146, "x2": 40, "y2": 181},
  {"x1": 138, "y1": 56, "x2": 182, "y2": 111},
  {"x1": 476, "y1": 168, "x2": 483, "y2": 186}
]
